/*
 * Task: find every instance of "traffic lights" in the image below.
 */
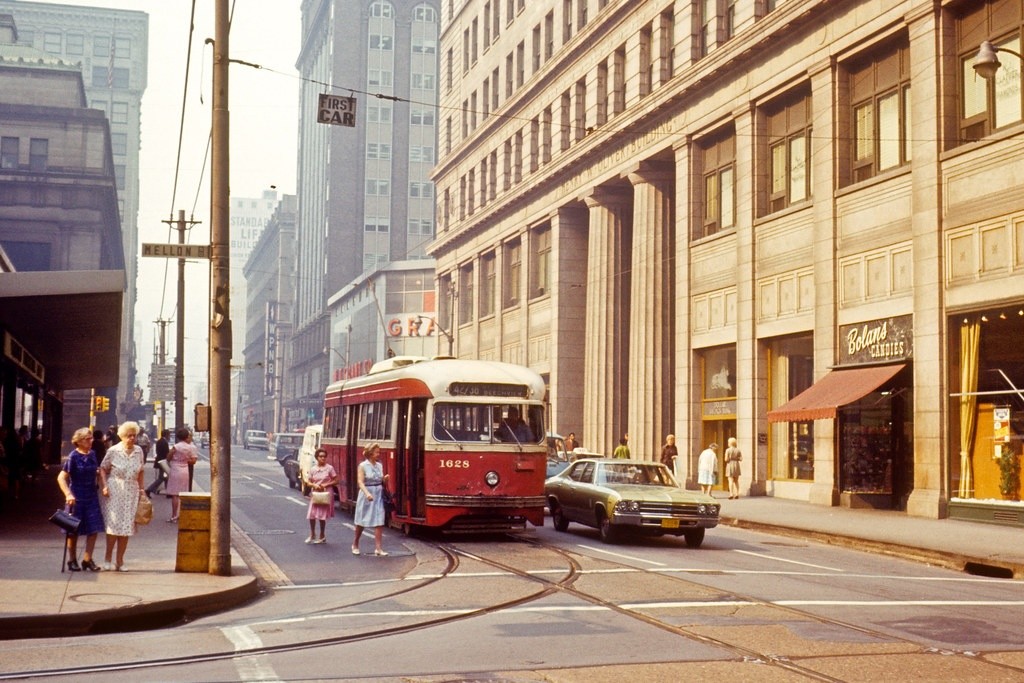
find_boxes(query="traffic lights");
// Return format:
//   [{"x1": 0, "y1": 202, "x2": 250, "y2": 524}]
[{"x1": 96, "y1": 397, "x2": 102, "y2": 411}]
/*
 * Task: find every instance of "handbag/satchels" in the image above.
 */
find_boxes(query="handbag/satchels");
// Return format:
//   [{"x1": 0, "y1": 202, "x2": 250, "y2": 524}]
[
  {"x1": 135, "y1": 490, "x2": 152, "y2": 525},
  {"x1": 158, "y1": 460, "x2": 170, "y2": 474},
  {"x1": 49, "y1": 509, "x2": 81, "y2": 534},
  {"x1": 312, "y1": 488, "x2": 330, "y2": 505},
  {"x1": 382, "y1": 481, "x2": 396, "y2": 512}
]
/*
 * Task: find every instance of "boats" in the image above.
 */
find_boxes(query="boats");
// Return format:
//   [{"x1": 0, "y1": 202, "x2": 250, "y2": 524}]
[{"x1": 103, "y1": 396, "x2": 109, "y2": 412}]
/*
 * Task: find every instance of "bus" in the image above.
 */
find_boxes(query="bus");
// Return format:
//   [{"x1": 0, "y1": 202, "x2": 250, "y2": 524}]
[{"x1": 319, "y1": 355, "x2": 545, "y2": 545}]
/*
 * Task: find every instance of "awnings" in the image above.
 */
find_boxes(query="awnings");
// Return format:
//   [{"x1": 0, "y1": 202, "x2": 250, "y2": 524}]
[{"x1": 766, "y1": 364, "x2": 907, "y2": 424}]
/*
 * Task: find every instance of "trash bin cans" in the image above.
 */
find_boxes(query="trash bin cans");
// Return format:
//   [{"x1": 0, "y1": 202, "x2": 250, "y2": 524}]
[{"x1": 175, "y1": 491, "x2": 212, "y2": 572}]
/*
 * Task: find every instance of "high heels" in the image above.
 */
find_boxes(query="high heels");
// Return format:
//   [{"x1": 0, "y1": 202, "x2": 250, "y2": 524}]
[
  {"x1": 375, "y1": 549, "x2": 388, "y2": 556},
  {"x1": 68, "y1": 561, "x2": 80, "y2": 571},
  {"x1": 82, "y1": 559, "x2": 100, "y2": 571},
  {"x1": 352, "y1": 544, "x2": 360, "y2": 554}
]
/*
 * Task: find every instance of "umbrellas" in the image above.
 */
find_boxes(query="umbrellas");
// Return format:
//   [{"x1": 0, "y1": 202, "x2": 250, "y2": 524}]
[{"x1": 545, "y1": 431, "x2": 563, "y2": 439}]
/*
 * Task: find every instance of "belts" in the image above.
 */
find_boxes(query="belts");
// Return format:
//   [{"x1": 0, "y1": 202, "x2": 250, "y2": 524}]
[{"x1": 365, "y1": 484, "x2": 381, "y2": 486}]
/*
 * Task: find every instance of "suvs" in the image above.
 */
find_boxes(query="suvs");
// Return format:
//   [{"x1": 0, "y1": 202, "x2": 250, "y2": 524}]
[{"x1": 243, "y1": 430, "x2": 270, "y2": 451}]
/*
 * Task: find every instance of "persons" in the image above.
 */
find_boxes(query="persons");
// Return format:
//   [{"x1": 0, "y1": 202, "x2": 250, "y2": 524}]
[
  {"x1": 351, "y1": 442, "x2": 390, "y2": 557},
  {"x1": 613, "y1": 433, "x2": 631, "y2": 459},
  {"x1": 144, "y1": 427, "x2": 199, "y2": 523},
  {"x1": 697, "y1": 443, "x2": 721, "y2": 499},
  {"x1": 304, "y1": 448, "x2": 340, "y2": 545},
  {"x1": 57, "y1": 427, "x2": 101, "y2": 572},
  {"x1": 564, "y1": 432, "x2": 579, "y2": 451},
  {"x1": 495, "y1": 406, "x2": 535, "y2": 442},
  {"x1": 659, "y1": 434, "x2": 678, "y2": 476},
  {"x1": 99, "y1": 420, "x2": 145, "y2": 572},
  {"x1": 724, "y1": 437, "x2": 743, "y2": 500},
  {"x1": 0, "y1": 424, "x2": 42, "y2": 498},
  {"x1": 91, "y1": 424, "x2": 152, "y2": 490}
]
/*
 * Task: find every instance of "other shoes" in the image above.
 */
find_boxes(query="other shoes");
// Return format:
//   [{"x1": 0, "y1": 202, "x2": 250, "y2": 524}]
[
  {"x1": 305, "y1": 537, "x2": 318, "y2": 543},
  {"x1": 728, "y1": 497, "x2": 733, "y2": 499},
  {"x1": 115, "y1": 564, "x2": 129, "y2": 571},
  {"x1": 166, "y1": 495, "x2": 172, "y2": 498},
  {"x1": 735, "y1": 496, "x2": 738, "y2": 499},
  {"x1": 105, "y1": 562, "x2": 111, "y2": 570},
  {"x1": 145, "y1": 489, "x2": 151, "y2": 498},
  {"x1": 315, "y1": 537, "x2": 326, "y2": 543},
  {"x1": 166, "y1": 516, "x2": 178, "y2": 523}
]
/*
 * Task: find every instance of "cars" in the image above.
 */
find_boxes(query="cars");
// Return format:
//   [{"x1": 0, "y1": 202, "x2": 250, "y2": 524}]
[
  {"x1": 557, "y1": 450, "x2": 627, "y2": 477},
  {"x1": 201, "y1": 432, "x2": 210, "y2": 448},
  {"x1": 285, "y1": 446, "x2": 299, "y2": 489},
  {"x1": 545, "y1": 456, "x2": 721, "y2": 546}
]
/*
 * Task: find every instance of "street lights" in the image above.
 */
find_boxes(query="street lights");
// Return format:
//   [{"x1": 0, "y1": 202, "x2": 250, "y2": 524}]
[
  {"x1": 414, "y1": 315, "x2": 454, "y2": 356},
  {"x1": 322, "y1": 345, "x2": 349, "y2": 379}
]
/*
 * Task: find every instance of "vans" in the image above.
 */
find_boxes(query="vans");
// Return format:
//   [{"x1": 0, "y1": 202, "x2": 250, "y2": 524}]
[
  {"x1": 275, "y1": 434, "x2": 305, "y2": 462},
  {"x1": 545, "y1": 432, "x2": 570, "y2": 515},
  {"x1": 299, "y1": 425, "x2": 324, "y2": 497}
]
